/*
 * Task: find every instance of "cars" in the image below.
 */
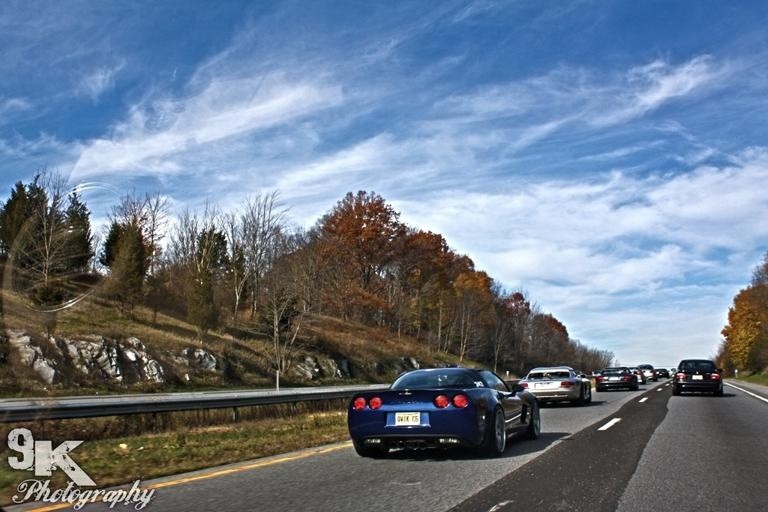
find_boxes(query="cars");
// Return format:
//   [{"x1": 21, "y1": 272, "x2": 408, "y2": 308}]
[
  {"x1": 671, "y1": 360, "x2": 723, "y2": 396},
  {"x1": 592, "y1": 364, "x2": 669, "y2": 392},
  {"x1": 519, "y1": 366, "x2": 592, "y2": 406}
]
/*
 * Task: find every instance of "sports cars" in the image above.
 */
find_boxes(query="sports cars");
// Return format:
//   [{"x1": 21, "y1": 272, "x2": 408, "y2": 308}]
[{"x1": 348, "y1": 368, "x2": 540, "y2": 457}]
